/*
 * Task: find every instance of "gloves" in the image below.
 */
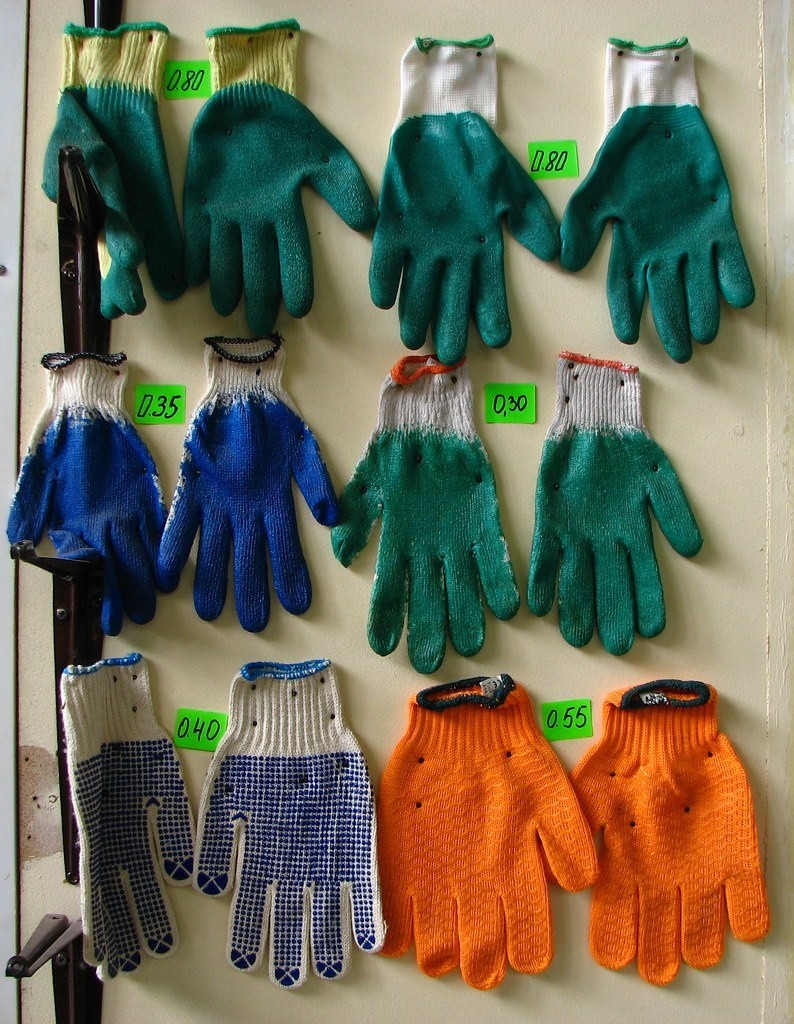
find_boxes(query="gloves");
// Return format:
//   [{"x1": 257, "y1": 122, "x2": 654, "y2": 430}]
[
  {"x1": 7, "y1": 352, "x2": 167, "y2": 637},
  {"x1": 331, "y1": 353, "x2": 520, "y2": 675},
  {"x1": 60, "y1": 653, "x2": 196, "y2": 982},
  {"x1": 193, "y1": 659, "x2": 388, "y2": 990},
  {"x1": 571, "y1": 678, "x2": 771, "y2": 986},
  {"x1": 528, "y1": 351, "x2": 705, "y2": 656},
  {"x1": 560, "y1": 36, "x2": 755, "y2": 364},
  {"x1": 183, "y1": 18, "x2": 380, "y2": 337},
  {"x1": 369, "y1": 33, "x2": 560, "y2": 366},
  {"x1": 376, "y1": 674, "x2": 599, "y2": 990},
  {"x1": 41, "y1": 21, "x2": 184, "y2": 320},
  {"x1": 160, "y1": 330, "x2": 342, "y2": 633}
]
